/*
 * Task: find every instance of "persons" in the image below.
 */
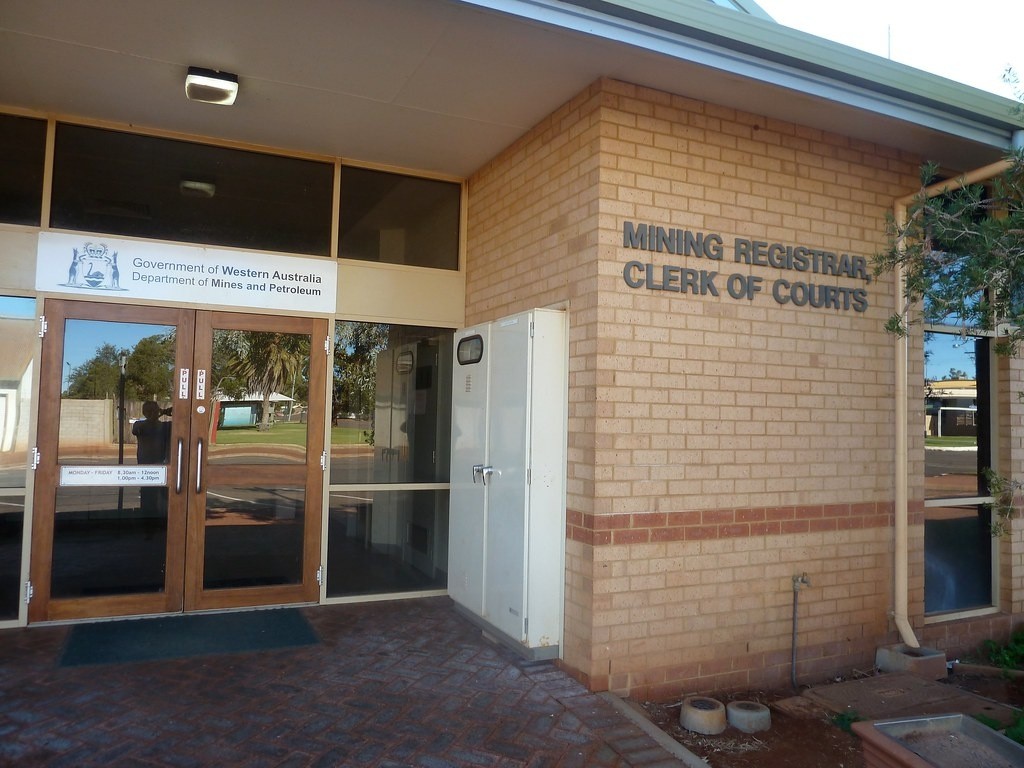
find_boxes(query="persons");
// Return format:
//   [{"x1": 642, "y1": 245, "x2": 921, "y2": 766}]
[{"x1": 132, "y1": 401, "x2": 172, "y2": 508}]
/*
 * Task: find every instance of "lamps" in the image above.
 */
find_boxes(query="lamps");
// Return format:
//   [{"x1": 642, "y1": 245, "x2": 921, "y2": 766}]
[{"x1": 185, "y1": 66, "x2": 239, "y2": 106}]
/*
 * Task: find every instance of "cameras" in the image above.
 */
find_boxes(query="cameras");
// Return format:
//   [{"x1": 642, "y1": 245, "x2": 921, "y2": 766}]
[{"x1": 160, "y1": 409, "x2": 171, "y2": 416}]
